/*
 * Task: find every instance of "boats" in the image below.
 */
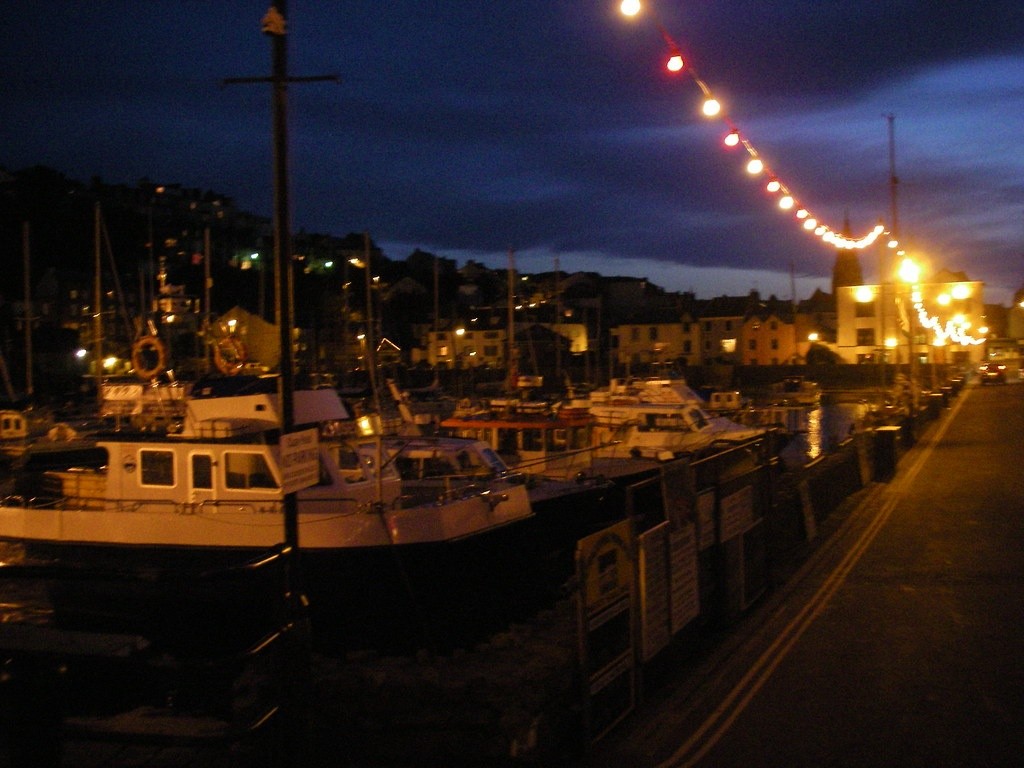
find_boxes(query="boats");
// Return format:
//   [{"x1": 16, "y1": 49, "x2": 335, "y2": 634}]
[{"x1": 0, "y1": 1, "x2": 974, "y2": 661}]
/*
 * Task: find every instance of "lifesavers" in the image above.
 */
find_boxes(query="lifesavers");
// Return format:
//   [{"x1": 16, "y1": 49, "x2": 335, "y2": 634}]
[
  {"x1": 214, "y1": 336, "x2": 248, "y2": 376},
  {"x1": 131, "y1": 336, "x2": 169, "y2": 381}
]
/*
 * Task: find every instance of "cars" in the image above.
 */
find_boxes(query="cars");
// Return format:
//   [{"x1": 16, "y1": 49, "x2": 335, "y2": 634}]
[{"x1": 978, "y1": 363, "x2": 1006, "y2": 385}]
